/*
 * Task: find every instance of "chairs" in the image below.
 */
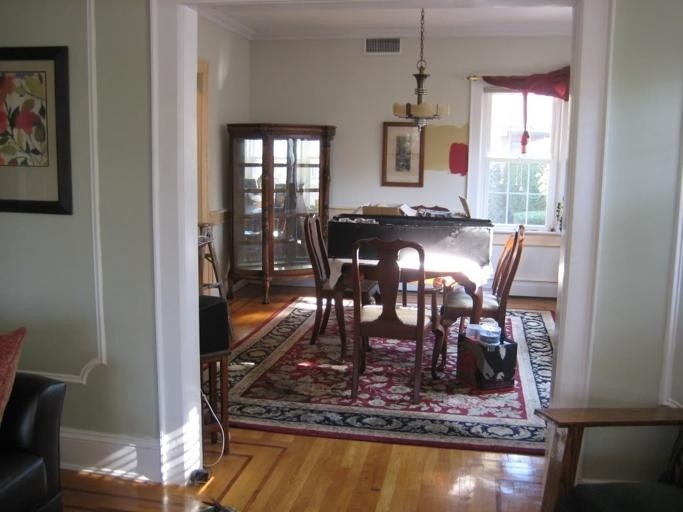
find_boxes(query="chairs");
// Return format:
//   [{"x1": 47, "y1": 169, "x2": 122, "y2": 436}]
[
  {"x1": 304, "y1": 205, "x2": 525, "y2": 405},
  {"x1": 0, "y1": 372, "x2": 66, "y2": 511}
]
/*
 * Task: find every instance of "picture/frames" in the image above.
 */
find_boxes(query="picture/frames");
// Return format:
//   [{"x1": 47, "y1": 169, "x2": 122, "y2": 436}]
[
  {"x1": 0, "y1": 46, "x2": 71, "y2": 215},
  {"x1": 382, "y1": 122, "x2": 424, "y2": 187}
]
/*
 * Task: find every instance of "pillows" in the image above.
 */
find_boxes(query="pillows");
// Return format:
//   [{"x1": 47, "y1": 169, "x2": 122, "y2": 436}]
[{"x1": 0, "y1": 330, "x2": 24, "y2": 426}]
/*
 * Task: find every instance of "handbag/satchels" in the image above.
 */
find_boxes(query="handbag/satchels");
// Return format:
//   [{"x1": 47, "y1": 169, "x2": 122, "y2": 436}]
[{"x1": 457, "y1": 330, "x2": 517, "y2": 388}]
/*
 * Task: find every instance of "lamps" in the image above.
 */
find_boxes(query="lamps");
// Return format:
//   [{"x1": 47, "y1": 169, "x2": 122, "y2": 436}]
[{"x1": 394, "y1": 9, "x2": 450, "y2": 129}]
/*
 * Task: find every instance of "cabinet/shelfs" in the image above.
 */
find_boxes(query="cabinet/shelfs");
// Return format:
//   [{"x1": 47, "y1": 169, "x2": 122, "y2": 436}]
[{"x1": 223, "y1": 124, "x2": 335, "y2": 306}]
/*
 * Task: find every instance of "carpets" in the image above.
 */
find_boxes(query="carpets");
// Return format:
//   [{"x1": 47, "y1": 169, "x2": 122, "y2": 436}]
[{"x1": 201, "y1": 294, "x2": 558, "y2": 457}]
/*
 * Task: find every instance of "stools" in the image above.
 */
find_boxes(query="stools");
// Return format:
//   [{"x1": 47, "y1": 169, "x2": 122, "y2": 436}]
[{"x1": 202, "y1": 351, "x2": 230, "y2": 456}]
